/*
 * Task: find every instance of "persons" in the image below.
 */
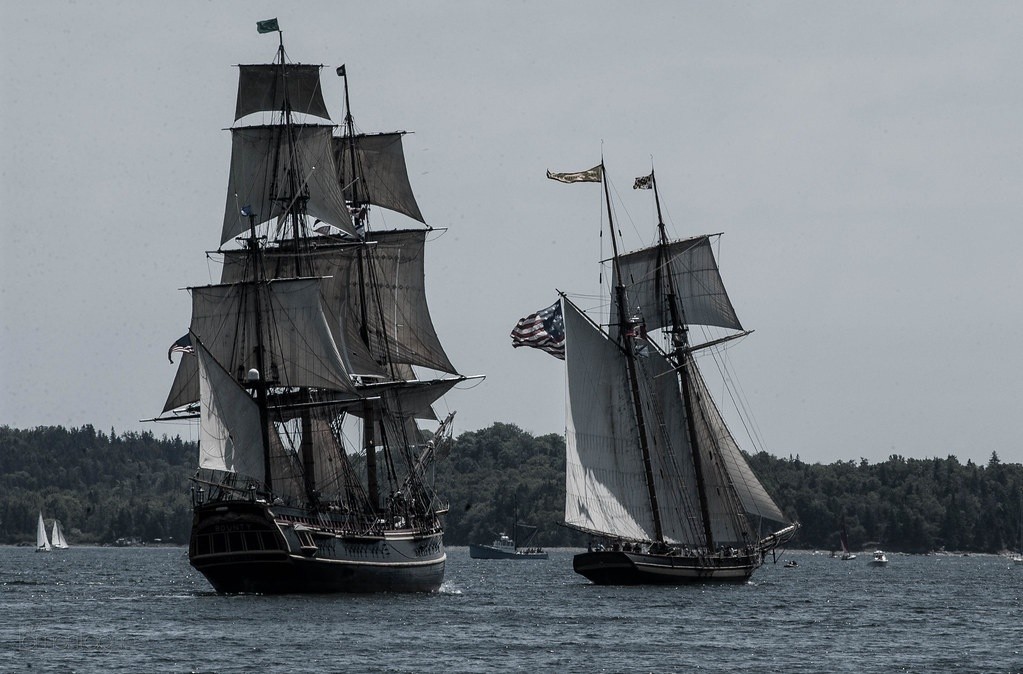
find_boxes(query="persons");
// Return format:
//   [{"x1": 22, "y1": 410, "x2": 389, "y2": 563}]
[
  {"x1": 514, "y1": 547, "x2": 543, "y2": 555},
  {"x1": 585, "y1": 538, "x2": 756, "y2": 556},
  {"x1": 225, "y1": 484, "x2": 434, "y2": 530}
]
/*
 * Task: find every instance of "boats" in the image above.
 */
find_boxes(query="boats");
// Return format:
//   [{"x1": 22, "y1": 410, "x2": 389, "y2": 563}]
[
  {"x1": 469, "y1": 490, "x2": 548, "y2": 558},
  {"x1": 784, "y1": 560, "x2": 799, "y2": 568},
  {"x1": 872, "y1": 550, "x2": 889, "y2": 567}
]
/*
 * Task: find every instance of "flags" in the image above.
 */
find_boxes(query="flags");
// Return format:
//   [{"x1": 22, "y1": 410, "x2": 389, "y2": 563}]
[
  {"x1": 256, "y1": 17, "x2": 279, "y2": 33},
  {"x1": 241, "y1": 205, "x2": 253, "y2": 216},
  {"x1": 509, "y1": 298, "x2": 566, "y2": 360},
  {"x1": 313, "y1": 226, "x2": 331, "y2": 236},
  {"x1": 336, "y1": 64, "x2": 346, "y2": 76},
  {"x1": 546, "y1": 164, "x2": 604, "y2": 183},
  {"x1": 39, "y1": 543, "x2": 45, "y2": 548},
  {"x1": 633, "y1": 173, "x2": 654, "y2": 190},
  {"x1": 168, "y1": 333, "x2": 196, "y2": 364}
]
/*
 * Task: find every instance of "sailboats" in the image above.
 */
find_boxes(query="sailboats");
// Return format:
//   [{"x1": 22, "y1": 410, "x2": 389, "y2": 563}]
[
  {"x1": 139, "y1": 17, "x2": 486, "y2": 594},
  {"x1": 52, "y1": 521, "x2": 69, "y2": 549},
  {"x1": 510, "y1": 139, "x2": 802, "y2": 585},
  {"x1": 35, "y1": 509, "x2": 53, "y2": 551}
]
